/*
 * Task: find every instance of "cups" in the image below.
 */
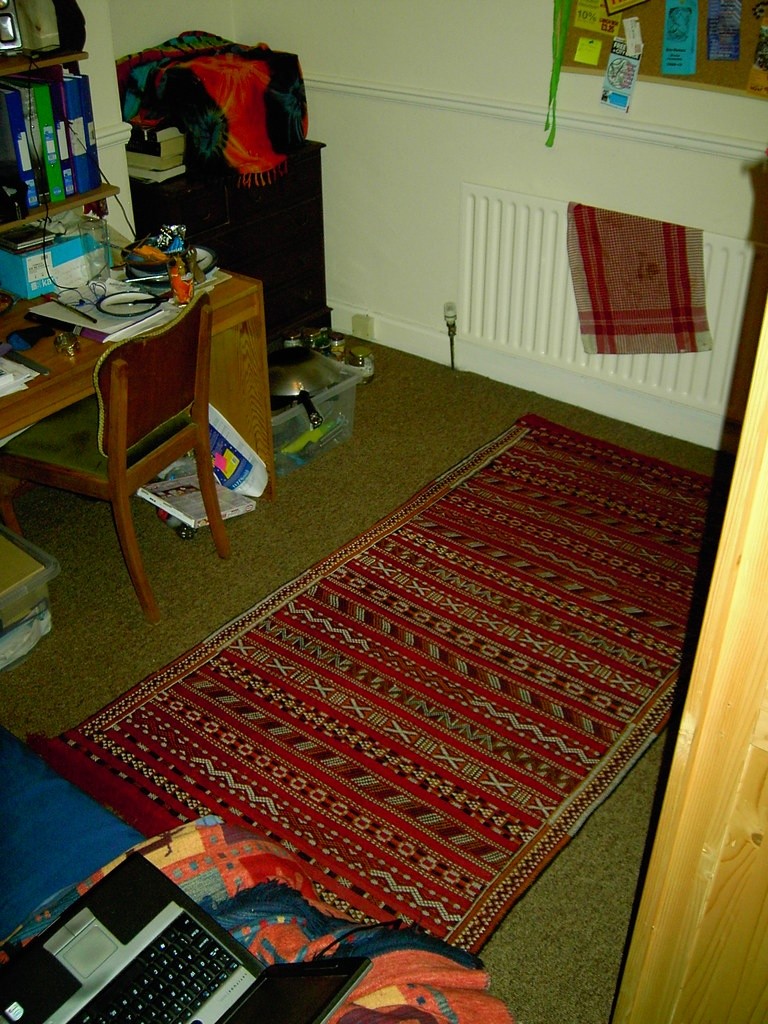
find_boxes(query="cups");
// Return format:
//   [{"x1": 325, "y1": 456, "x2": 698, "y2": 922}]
[{"x1": 78, "y1": 218, "x2": 111, "y2": 285}]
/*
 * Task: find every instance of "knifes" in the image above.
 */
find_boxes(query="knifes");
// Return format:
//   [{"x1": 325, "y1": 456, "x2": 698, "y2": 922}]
[{"x1": 106, "y1": 297, "x2": 170, "y2": 305}]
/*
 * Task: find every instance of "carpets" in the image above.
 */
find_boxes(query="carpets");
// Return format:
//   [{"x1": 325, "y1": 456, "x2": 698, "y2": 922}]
[{"x1": 22, "y1": 412, "x2": 712, "y2": 962}]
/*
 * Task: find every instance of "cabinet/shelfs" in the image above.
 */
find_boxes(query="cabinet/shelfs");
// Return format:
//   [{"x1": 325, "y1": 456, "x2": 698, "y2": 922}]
[
  {"x1": 0, "y1": 0, "x2": 123, "y2": 236},
  {"x1": 128, "y1": 137, "x2": 331, "y2": 366}
]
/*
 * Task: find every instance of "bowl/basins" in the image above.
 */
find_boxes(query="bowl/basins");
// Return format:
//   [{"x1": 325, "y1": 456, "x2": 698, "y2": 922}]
[{"x1": 120, "y1": 237, "x2": 192, "y2": 275}]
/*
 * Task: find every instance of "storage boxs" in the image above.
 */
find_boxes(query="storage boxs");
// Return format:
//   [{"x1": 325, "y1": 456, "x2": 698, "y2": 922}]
[
  {"x1": 0, "y1": 523, "x2": 63, "y2": 674},
  {"x1": 264, "y1": 345, "x2": 363, "y2": 479}
]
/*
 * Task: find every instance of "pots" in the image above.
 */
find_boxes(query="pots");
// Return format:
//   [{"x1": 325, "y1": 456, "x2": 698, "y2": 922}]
[{"x1": 266, "y1": 346, "x2": 339, "y2": 428}]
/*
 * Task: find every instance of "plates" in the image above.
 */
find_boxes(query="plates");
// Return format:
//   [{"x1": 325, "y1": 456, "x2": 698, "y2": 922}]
[
  {"x1": 124, "y1": 245, "x2": 218, "y2": 288},
  {"x1": 96, "y1": 291, "x2": 162, "y2": 318}
]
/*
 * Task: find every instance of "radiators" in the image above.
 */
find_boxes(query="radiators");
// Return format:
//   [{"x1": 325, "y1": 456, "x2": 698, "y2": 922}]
[{"x1": 453, "y1": 178, "x2": 767, "y2": 426}]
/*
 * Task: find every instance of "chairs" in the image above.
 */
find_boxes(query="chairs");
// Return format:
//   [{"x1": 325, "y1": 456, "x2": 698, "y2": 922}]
[{"x1": 0, "y1": 285, "x2": 233, "y2": 629}]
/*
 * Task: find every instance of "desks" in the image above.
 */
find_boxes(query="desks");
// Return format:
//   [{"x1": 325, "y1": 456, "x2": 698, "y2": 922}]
[{"x1": 0, "y1": 264, "x2": 278, "y2": 502}]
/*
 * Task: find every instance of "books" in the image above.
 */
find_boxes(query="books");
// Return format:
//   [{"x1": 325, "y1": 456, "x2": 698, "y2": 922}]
[
  {"x1": 22, "y1": 276, "x2": 171, "y2": 344},
  {"x1": 123, "y1": 125, "x2": 188, "y2": 185},
  {"x1": 0, "y1": 356, "x2": 42, "y2": 397},
  {"x1": 0, "y1": 223, "x2": 58, "y2": 255}
]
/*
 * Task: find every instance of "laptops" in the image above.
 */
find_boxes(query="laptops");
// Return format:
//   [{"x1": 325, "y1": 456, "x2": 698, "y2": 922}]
[{"x1": 0, "y1": 850, "x2": 373, "y2": 1024}]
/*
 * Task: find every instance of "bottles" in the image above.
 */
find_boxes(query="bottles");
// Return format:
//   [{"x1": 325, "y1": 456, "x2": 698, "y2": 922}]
[
  {"x1": 321, "y1": 346, "x2": 340, "y2": 403},
  {"x1": 155, "y1": 505, "x2": 199, "y2": 540}
]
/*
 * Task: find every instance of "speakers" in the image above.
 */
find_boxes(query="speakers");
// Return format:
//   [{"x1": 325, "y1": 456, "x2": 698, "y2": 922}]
[{"x1": 0, "y1": 0, "x2": 22, "y2": 50}]
